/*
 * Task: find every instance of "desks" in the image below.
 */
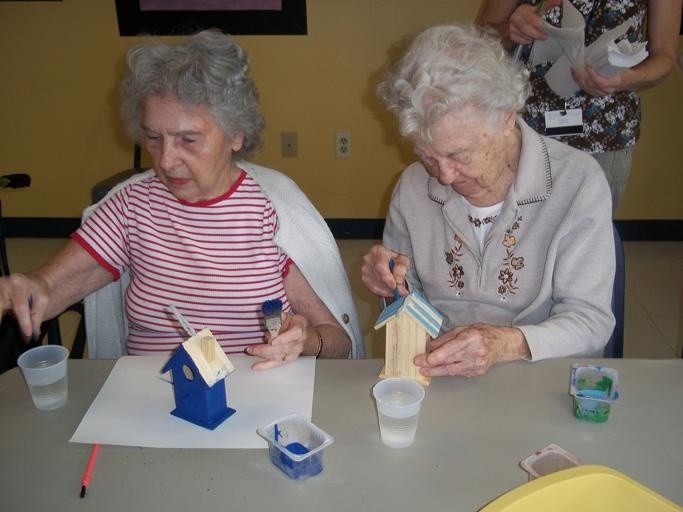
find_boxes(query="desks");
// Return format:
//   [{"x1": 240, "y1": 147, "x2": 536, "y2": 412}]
[{"x1": 0, "y1": 357, "x2": 683, "y2": 512}]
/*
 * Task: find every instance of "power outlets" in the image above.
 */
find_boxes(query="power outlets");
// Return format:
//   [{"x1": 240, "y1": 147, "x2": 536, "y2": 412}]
[{"x1": 336, "y1": 132, "x2": 351, "y2": 160}]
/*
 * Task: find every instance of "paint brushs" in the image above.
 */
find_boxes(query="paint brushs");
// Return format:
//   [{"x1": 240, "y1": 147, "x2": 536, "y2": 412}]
[
  {"x1": 513, "y1": 0, "x2": 546, "y2": 63},
  {"x1": 80, "y1": 442, "x2": 99, "y2": 498}
]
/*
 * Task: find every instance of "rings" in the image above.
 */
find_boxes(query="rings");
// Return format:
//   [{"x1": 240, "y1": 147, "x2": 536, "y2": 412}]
[{"x1": 281, "y1": 352, "x2": 288, "y2": 360}]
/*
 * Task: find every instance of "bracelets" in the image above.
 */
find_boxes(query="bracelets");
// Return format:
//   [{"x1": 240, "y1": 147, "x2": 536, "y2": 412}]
[{"x1": 308, "y1": 322, "x2": 326, "y2": 360}]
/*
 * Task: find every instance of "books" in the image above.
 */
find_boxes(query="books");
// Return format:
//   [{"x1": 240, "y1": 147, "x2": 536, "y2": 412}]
[{"x1": 528, "y1": 0, "x2": 650, "y2": 98}]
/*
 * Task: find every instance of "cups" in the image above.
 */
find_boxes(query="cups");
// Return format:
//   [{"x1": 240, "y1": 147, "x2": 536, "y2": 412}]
[
  {"x1": 372, "y1": 379, "x2": 425, "y2": 451},
  {"x1": 18, "y1": 344, "x2": 71, "y2": 410}
]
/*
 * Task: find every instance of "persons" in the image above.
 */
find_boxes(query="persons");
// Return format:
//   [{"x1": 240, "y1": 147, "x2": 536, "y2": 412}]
[
  {"x1": 0, "y1": 29, "x2": 365, "y2": 369},
  {"x1": 477, "y1": 0, "x2": 682, "y2": 220},
  {"x1": 361, "y1": 24, "x2": 619, "y2": 378}
]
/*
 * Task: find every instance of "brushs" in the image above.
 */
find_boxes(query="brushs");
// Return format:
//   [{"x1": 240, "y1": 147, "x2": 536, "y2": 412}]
[
  {"x1": 388, "y1": 258, "x2": 399, "y2": 301},
  {"x1": 261, "y1": 299, "x2": 283, "y2": 343}
]
